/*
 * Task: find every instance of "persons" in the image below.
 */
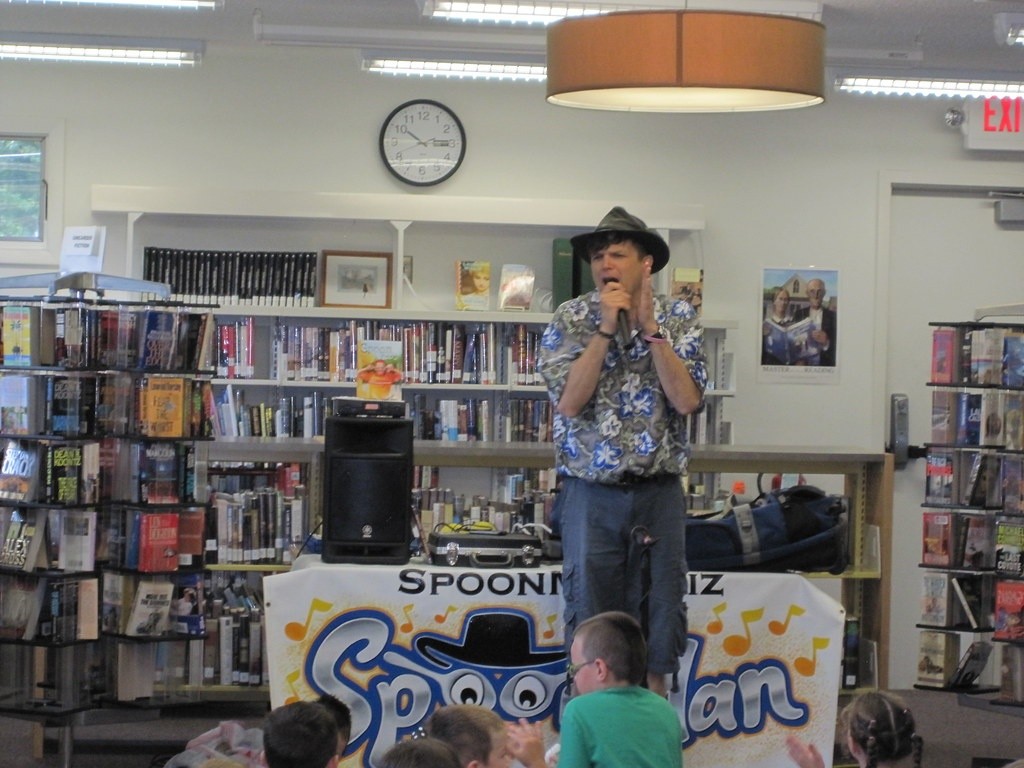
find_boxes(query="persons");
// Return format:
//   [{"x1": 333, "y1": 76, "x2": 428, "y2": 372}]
[
  {"x1": 763, "y1": 278, "x2": 837, "y2": 368},
  {"x1": 552, "y1": 611, "x2": 683, "y2": 768},
  {"x1": 260, "y1": 702, "x2": 338, "y2": 768},
  {"x1": 787, "y1": 690, "x2": 923, "y2": 768},
  {"x1": 537, "y1": 204, "x2": 707, "y2": 698},
  {"x1": 462, "y1": 263, "x2": 490, "y2": 310},
  {"x1": 374, "y1": 702, "x2": 547, "y2": 768}
]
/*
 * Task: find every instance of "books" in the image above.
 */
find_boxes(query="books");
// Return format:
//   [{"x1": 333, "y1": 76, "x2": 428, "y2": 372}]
[
  {"x1": 497, "y1": 263, "x2": 536, "y2": 313},
  {"x1": 765, "y1": 317, "x2": 820, "y2": 365},
  {"x1": 0, "y1": 296, "x2": 553, "y2": 691},
  {"x1": 683, "y1": 403, "x2": 711, "y2": 444},
  {"x1": 456, "y1": 260, "x2": 490, "y2": 310},
  {"x1": 914, "y1": 325, "x2": 1024, "y2": 703},
  {"x1": 141, "y1": 246, "x2": 317, "y2": 306}
]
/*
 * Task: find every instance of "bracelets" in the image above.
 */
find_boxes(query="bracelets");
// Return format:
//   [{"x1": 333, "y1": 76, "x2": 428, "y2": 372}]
[
  {"x1": 641, "y1": 332, "x2": 665, "y2": 345},
  {"x1": 598, "y1": 330, "x2": 614, "y2": 339}
]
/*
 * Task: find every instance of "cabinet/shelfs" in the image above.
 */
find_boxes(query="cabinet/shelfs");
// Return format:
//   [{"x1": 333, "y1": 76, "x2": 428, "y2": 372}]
[
  {"x1": 0, "y1": 276, "x2": 223, "y2": 768},
  {"x1": 913, "y1": 321, "x2": 1024, "y2": 712},
  {"x1": 87, "y1": 192, "x2": 892, "y2": 699}
]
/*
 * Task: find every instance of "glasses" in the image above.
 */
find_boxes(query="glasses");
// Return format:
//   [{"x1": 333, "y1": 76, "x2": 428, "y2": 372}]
[{"x1": 567, "y1": 660, "x2": 594, "y2": 678}]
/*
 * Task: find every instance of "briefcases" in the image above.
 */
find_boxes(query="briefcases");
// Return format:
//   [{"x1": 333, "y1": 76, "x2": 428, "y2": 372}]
[{"x1": 427, "y1": 531, "x2": 543, "y2": 567}]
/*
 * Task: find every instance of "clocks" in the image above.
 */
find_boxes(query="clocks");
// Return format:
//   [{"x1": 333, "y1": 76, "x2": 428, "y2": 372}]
[{"x1": 378, "y1": 99, "x2": 468, "y2": 187}]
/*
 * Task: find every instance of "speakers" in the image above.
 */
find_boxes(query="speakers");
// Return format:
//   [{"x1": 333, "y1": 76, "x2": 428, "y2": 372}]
[{"x1": 325, "y1": 415, "x2": 414, "y2": 566}]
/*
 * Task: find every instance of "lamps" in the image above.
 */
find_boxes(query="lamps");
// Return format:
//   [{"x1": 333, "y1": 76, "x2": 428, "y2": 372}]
[
  {"x1": 362, "y1": 59, "x2": 549, "y2": 84},
  {"x1": 828, "y1": 45, "x2": 925, "y2": 66},
  {"x1": 419, "y1": 1, "x2": 613, "y2": 27},
  {"x1": 545, "y1": 9, "x2": 827, "y2": 113},
  {"x1": 992, "y1": 12, "x2": 1024, "y2": 46},
  {"x1": 1, "y1": 31, "x2": 208, "y2": 66},
  {"x1": 832, "y1": 69, "x2": 1024, "y2": 97},
  {"x1": 254, "y1": 14, "x2": 548, "y2": 56},
  {"x1": 943, "y1": 106, "x2": 966, "y2": 129}
]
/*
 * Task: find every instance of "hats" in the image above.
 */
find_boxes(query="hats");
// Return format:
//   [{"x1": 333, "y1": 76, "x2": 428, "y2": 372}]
[{"x1": 570, "y1": 207, "x2": 670, "y2": 274}]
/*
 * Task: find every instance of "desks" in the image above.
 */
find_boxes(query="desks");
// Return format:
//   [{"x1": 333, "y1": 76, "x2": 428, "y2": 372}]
[{"x1": 264, "y1": 569, "x2": 847, "y2": 765}]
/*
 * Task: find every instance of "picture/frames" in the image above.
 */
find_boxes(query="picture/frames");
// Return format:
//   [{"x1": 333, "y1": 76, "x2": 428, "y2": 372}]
[{"x1": 321, "y1": 250, "x2": 393, "y2": 308}]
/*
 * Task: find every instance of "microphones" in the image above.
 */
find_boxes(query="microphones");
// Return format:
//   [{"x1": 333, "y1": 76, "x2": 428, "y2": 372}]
[{"x1": 619, "y1": 309, "x2": 637, "y2": 350}]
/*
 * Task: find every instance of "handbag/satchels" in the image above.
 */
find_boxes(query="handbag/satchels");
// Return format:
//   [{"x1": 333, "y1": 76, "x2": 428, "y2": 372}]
[{"x1": 685, "y1": 485, "x2": 849, "y2": 571}]
